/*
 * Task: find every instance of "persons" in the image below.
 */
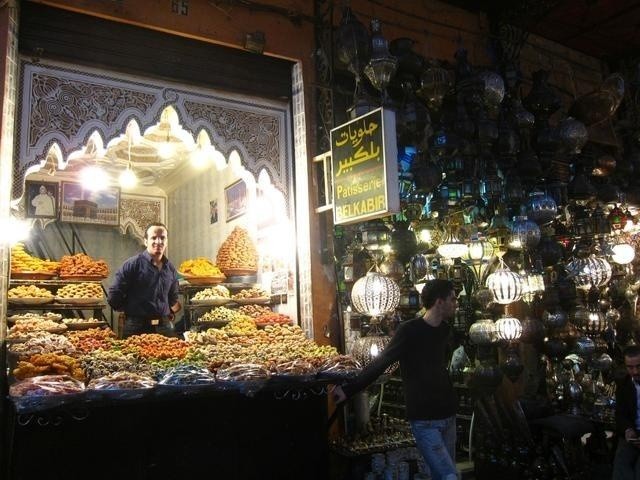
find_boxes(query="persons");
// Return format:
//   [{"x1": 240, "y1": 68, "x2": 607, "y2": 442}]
[
  {"x1": 611, "y1": 345, "x2": 640, "y2": 480},
  {"x1": 330, "y1": 278, "x2": 462, "y2": 479},
  {"x1": 107, "y1": 222, "x2": 181, "y2": 339}
]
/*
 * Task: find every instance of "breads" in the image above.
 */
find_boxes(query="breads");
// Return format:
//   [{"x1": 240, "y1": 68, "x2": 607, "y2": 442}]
[
  {"x1": 216, "y1": 226, "x2": 258, "y2": 269},
  {"x1": 8, "y1": 243, "x2": 109, "y2": 298}
]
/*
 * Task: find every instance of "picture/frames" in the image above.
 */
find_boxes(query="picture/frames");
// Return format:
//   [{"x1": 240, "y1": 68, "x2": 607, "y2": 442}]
[
  {"x1": 23, "y1": 180, "x2": 122, "y2": 228},
  {"x1": 223, "y1": 176, "x2": 248, "y2": 224}
]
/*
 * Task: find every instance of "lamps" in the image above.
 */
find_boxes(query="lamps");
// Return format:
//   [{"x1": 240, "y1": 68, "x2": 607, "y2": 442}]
[
  {"x1": 77, "y1": 112, "x2": 231, "y2": 194},
  {"x1": 342, "y1": 6, "x2": 451, "y2": 112},
  {"x1": 569, "y1": 194, "x2": 637, "y2": 264}
]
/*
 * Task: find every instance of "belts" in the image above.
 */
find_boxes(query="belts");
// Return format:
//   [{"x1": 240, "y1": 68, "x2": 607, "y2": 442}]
[{"x1": 133, "y1": 318, "x2": 168, "y2": 325}]
